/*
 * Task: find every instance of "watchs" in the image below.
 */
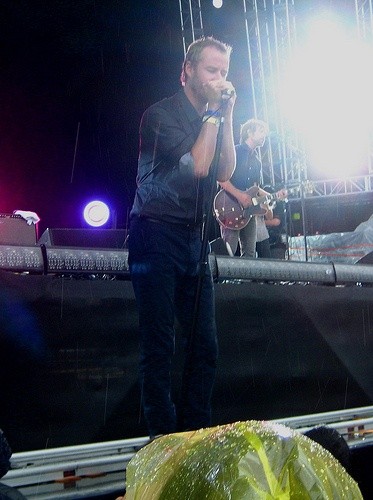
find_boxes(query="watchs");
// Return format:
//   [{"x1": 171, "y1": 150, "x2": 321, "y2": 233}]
[{"x1": 202, "y1": 116, "x2": 221, "y2": 128}]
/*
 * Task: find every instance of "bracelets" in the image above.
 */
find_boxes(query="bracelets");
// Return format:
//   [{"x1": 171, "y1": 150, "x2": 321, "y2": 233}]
[{"x1": 202, "y1": 110, "x2": 222, "y2": 116}]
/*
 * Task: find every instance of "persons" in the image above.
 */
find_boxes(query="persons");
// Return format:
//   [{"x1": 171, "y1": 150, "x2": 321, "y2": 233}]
[
  {"x1": 126, "y1": 37, "x2": 237, "y2": 451},
  {"x1": 0, "y1": 430, "x2": 31, "y2": 500},
  {"x1": 256, "y1": 203, "x2": 273, "y2": 259},
  {"x1": 216, "y1": 119, "x2": 289, "y2": 283},
  {"x1": 301, "y1": 425, "x2": 356, "y2": 479},
  {"x1": 114, "y1": 420, "x2": 363, "y2": 500},
  {"x1": 262, "y1": 185, "x2": 289, "y2": 259}
]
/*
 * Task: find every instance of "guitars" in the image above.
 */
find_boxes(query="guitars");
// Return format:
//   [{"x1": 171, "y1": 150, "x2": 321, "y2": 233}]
[{"x1": 212, "y1": 182, "x2": 314, "y2": 231}]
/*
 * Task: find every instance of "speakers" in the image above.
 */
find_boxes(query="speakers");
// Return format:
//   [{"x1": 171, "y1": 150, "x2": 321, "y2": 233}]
[
  {"x1": 0, "y1": 213, "x2": 37, "y2": 247},
  {"x1": 37, "y1": 228, "x2": 130, "y2": 249}
]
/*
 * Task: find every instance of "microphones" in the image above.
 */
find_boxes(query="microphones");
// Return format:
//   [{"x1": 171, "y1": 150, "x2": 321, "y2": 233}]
[{"x1": 221, "y1": 88, "x2": 235, "y2": 96}]
